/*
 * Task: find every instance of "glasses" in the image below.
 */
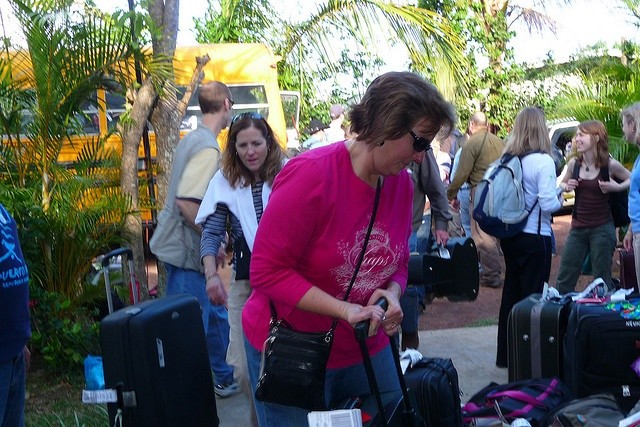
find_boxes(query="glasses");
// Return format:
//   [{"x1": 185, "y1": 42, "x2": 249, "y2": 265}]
[
  {"x1": 231, "y1": 112, "x2": 263, "y2": 124},
  {"x1": 410, "y1": 128, "x2": 431, "y2": 152}
]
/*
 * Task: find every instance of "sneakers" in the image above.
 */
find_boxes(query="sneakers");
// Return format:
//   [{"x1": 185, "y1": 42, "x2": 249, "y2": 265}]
[
  {"x1": 480, "y1": 274, "x2": 502, "y2": 288},
  {"x1": 402, "y1": 332, "x2": 418, "y2": 354},
  {"x1": 214, "y1": 379, "x2": 244, "y2": 399}
]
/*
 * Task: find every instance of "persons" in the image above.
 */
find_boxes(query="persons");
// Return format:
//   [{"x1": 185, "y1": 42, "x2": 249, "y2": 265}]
[
  {"x1": 148, "y1": 80, "x2": 242, "y2": 398},
  {"x1": 556, "y1": 121, "x2": 631, "y2": 295},
  {"x1": 303, "y1": 103, "x2": 358, "y2": 150},
  {"x1": 400, "y1": 147, "x2": 453, "y2": 351},
  {"x1": 194, "y1": 112, "x2": 292, "y2": 427},
  {"x1": 622, "y1": 103, "x2": 639, "y2": 296},
  {"x1": 0, "y1": 204, "x2": 32, "y2": 427},
  {"x1": 472, "y1": 108, "x2": 565, "y2": 369},
  {"x1": 447, "y1": 111, "x2": 506, "y2": 289},
  {"x1": 242, "y1": 71, "x2": 455, "y2": 427},
  {"x1": 450, "y1": 147, "x2": 471, "y2": 239}
]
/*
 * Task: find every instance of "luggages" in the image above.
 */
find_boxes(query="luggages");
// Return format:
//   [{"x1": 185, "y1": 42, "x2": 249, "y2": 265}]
[
  {"x1": 564, "y1": 291, "x2": 640, "y2": 398},
  {"x1": 507, "y1": 291, "x2": 571, "y2": 382},
  {"x1": 99, "y1": 247, "x2": 219, "y2": 427},
  {"x1": 610, "y1": 247, "x2": 639, "y2": 297},
  {"x1": 333, "y1": 297, "x2": 425, "y2": 427},
  {"x1": 403, "y1": 357, "x2": 462, "y2": 426}
]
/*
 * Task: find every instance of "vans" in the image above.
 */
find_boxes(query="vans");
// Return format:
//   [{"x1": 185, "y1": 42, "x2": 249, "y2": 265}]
[{"x1": 1, "y1": 42, "x2": 287, "y2": 232}]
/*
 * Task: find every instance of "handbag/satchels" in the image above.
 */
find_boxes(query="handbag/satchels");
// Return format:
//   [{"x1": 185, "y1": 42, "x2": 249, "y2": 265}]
[
  {"x1": 540, "y1": 394, "x2": 624, "y2": 427},
  {"x1": 460, "y1": 377, "x2": 572, "y2": 427},
  {"x1": 256, "y1": 319, "x2": 333, "y2": 412}
]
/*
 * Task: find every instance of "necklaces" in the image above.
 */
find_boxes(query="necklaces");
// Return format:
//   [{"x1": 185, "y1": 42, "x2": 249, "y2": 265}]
[{"x1": 583, "y1": 159, "x2": 592, "y2": 172}]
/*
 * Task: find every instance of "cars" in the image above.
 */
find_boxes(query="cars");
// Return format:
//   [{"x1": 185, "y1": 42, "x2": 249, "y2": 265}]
[{"x1": 548, "y1": 120, "x2": 583, "y2": 208}]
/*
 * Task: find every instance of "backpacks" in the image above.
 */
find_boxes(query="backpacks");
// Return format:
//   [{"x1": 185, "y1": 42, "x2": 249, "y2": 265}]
[
  {"x1": 471, "y1": 148, "x2": 546, "y2": 240},
  {"x1": 572, "y1": 158, "x2": 630, "y2": 228}
]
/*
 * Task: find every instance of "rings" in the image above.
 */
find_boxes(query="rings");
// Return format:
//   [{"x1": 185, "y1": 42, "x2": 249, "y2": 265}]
[
  {"x1": 382, "y1": 317, "x2": 386, "y2": 321},
  {"x1": 391, "y1": 321, "x2": 397, "y2": 329}
]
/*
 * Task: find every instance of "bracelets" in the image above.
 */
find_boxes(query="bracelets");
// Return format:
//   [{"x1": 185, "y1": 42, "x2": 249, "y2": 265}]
[
  {"x1": 565, "y1": 186, "x2": 567, "y2": 192},
  {"x1": 206, "y1": 272, "x2": 219, "y2": 280}
]
